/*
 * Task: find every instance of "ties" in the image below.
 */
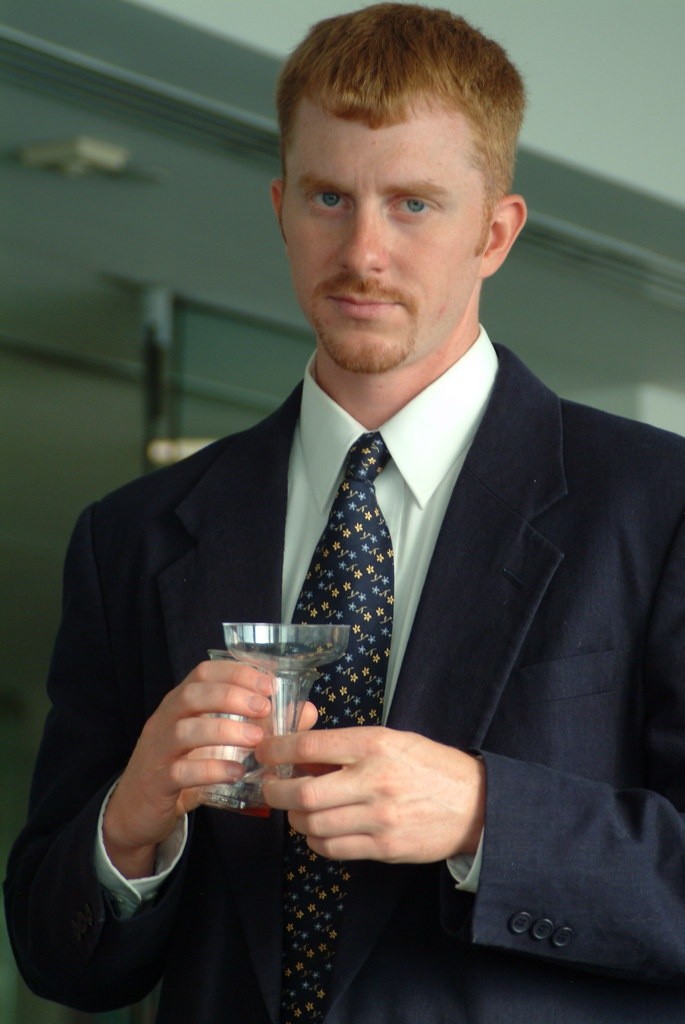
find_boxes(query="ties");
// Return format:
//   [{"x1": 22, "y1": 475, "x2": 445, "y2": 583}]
[{"x1": 276, "y1": 433, "x2": 396, "y2": 1024}]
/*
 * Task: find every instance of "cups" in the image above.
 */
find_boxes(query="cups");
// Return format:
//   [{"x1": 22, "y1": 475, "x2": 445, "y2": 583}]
[{"x1": 182, "y1": 649, "x2": 265, "y2": 811}]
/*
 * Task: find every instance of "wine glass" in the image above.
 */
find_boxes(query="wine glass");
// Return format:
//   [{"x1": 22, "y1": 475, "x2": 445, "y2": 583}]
[{"x1": 224, "y1": 622, "x2": 351, "y2": 787}]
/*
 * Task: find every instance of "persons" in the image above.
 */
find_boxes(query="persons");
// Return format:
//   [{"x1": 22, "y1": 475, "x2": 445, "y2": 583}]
[{"x1": 3, "y1": 2, "x2": 685, "y2": 1024}]
